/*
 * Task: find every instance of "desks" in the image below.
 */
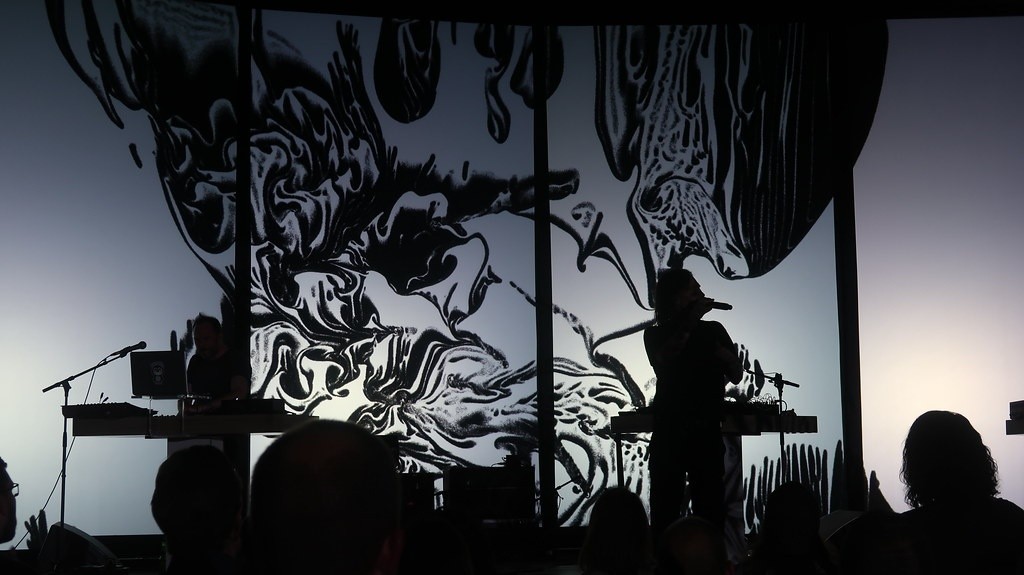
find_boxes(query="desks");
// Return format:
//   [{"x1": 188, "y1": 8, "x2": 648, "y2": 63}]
[
  {"x1": 74, "y1": 415, "x2": 319, "y2": 517},
  {"x1": 612, "y1": 414, "x2": 817, "y2": 566}
]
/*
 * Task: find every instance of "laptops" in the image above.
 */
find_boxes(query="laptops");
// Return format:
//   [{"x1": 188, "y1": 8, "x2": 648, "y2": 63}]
[{"x1": 130, "y1": 352, "x2": 211, "y2": 400}]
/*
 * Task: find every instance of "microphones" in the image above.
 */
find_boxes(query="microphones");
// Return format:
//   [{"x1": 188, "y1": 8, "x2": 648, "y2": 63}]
[
  {"x1": 111, "y1": 342, "x2": 147, "y2": 355},
  {"x1": 710, "y1": 302, "x2": 732, "y2": 310}
]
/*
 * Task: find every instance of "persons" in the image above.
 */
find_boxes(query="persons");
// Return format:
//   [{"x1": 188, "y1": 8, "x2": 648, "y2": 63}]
[
  {"x1": 187, "y1": 316, "x2": 251, "y2": 513},
  {"x1": 0, "y1": 458, "x2": 48, "y2": 575},
  {"x1": 645, "y1": 268, "x2": 743, "y2": 537},
  {"x1": 152, "y1": 411, "x2": 1024, "y2": 575}
]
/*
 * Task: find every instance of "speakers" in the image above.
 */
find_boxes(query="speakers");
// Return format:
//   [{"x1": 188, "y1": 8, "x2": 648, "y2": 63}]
[
  {"x1": 817, "y1": 510, "x2": 873, "y2": 568},
  {"x1": 37, "y1": 521, "x2": 117, "y2": 568},
  {"x1": 397, "y1": 467, "x2": 547, "y2": 571}
]
